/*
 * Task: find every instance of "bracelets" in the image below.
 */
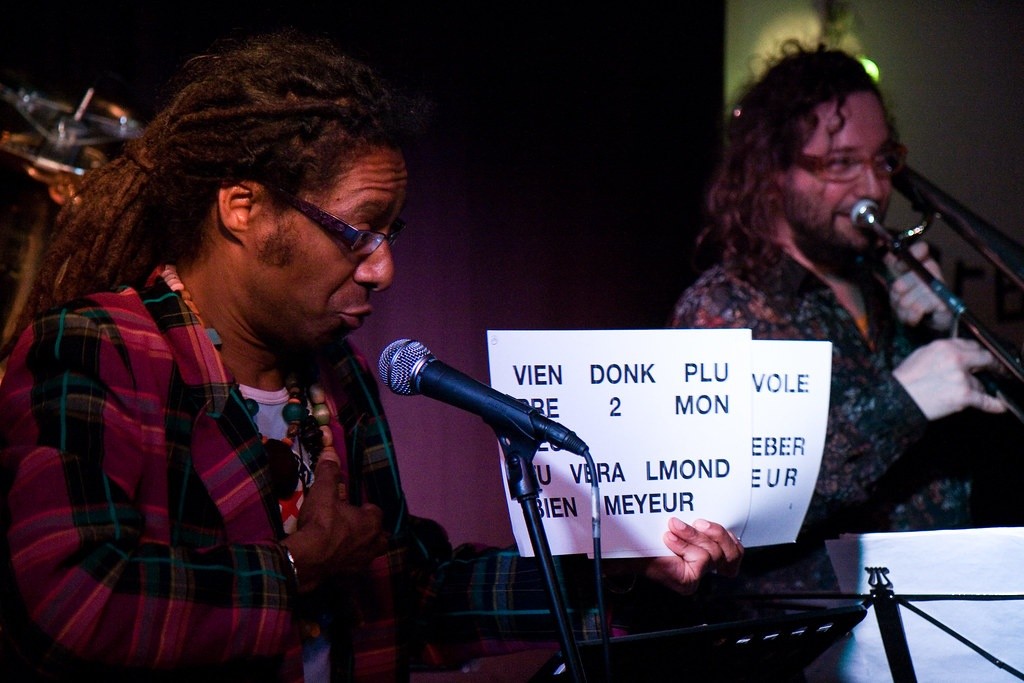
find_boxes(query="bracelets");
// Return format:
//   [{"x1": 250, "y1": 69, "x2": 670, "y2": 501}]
[{"x1": 283, "y1": 544, "x2": 302, "y2": 589}]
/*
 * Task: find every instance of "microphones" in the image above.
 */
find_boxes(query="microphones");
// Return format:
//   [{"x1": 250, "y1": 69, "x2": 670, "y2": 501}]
[{"x1": 376, "y1": 338, "x2": 588, "y2": 456}]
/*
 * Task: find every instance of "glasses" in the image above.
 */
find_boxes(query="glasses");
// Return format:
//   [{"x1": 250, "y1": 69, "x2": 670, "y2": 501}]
[
  {"x1": 253, "y1": 176, "x2": 408, "y2": 257},
  {"x1": 786, "y1": 142, "x2": 907, "y2": 185}
]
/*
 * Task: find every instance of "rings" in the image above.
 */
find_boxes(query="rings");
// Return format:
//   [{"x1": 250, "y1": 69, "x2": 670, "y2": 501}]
[{"x1": 711, "y1": 568, "x2": 719, "y2": 577}]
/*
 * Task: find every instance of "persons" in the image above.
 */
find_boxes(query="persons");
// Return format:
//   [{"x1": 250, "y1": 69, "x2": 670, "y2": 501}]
[
  {"x1": 0, "y1": 34, "x2": 745, "y2": 683},
  {"x1": 668, "y1": 37, "x2": 1009, "y2": 597}
]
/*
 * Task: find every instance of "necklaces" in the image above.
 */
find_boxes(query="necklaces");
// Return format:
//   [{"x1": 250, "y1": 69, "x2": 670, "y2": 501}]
[{"x1": 157, "y1": 258, "x2": 347, "y2": 641}]
